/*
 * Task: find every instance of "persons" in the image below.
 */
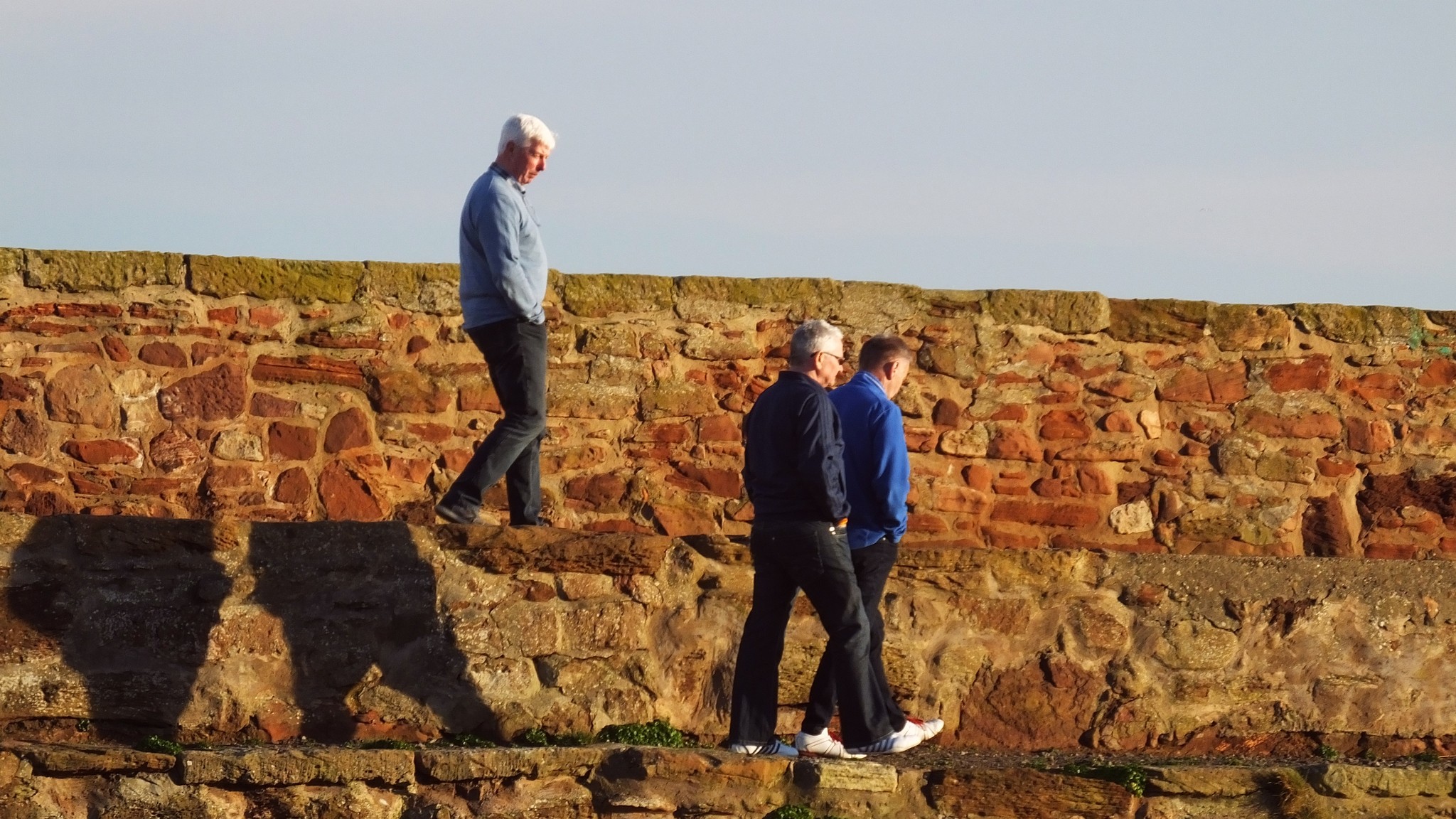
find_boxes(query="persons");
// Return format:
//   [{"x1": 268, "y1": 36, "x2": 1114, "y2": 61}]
[
  {"x1": 793, "y1": 329, "x2": 942, "y2": 756},
  {"x1": 430, "y1": 112, "x2": 562, "y2": 526},
  {"x1": 728, "y1": 316, "x2": 926, "y2": 760}
]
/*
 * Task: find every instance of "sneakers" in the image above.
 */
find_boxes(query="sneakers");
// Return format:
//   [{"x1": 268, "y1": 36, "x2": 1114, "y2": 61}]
[
  {"x1": 891, "y1": 719, "x2": 944, "y2": 741},
  {"x1": 795, "y1": 728, "x2": 867, "y2": 759},
  {"x1": 846, "y1": 719, "x2": 922, "y2": 754},
  {"x1": 731, "y1": 739, "x2": 800, "y2": 757}
]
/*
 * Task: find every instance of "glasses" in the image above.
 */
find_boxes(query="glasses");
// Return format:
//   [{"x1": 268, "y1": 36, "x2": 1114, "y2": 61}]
[{"x1": 812, "y1": 351, "x2": 849, "y2": 365}]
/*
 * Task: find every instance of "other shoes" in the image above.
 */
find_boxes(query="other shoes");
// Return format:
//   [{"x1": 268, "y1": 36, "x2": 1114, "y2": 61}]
[{"x1": 434, "y1": 502, "x2": 479, "y2": 522}]
